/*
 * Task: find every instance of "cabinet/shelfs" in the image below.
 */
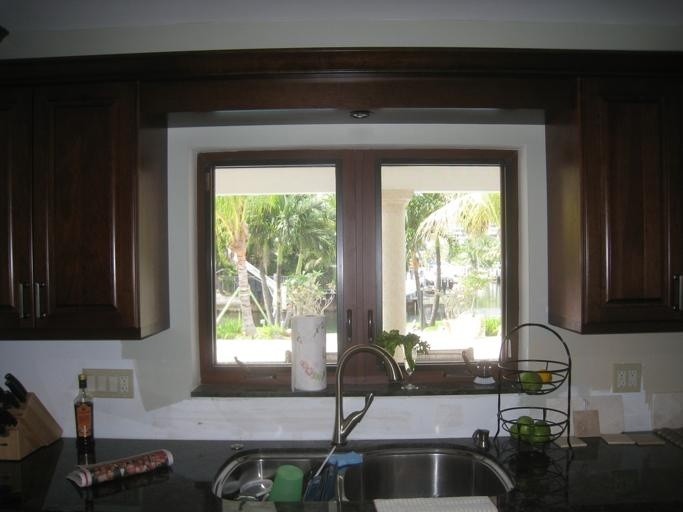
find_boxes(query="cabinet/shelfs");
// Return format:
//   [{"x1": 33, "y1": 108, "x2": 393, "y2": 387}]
[
  {"x1": 0, "y1": 80, "x2": 169, "y2": 341},
  {"x1": 544, "y1": 64, "x2": 683, "y2": 334}
]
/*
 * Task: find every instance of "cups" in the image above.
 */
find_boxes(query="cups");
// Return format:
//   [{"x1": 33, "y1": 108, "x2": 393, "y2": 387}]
[{"x1": 270, "y1": 464, "x2": 303, "y2": 502}]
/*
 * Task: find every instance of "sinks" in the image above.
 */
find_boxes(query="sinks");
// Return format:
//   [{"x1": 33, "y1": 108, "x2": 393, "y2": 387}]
[
  {"x1": 342, "y1": 441, "x2": 514, "y2": 502},
  {"x1": 209, "y1": 446, "x2": 342, "y2": 503}
]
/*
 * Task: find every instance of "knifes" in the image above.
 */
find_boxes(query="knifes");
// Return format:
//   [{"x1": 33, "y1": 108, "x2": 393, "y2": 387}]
[{"x1": 0, "y1": 372, "x2": 28, "y2": 428}]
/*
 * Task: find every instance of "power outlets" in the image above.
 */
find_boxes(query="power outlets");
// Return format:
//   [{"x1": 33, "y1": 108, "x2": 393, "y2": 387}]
[
  {"x1": 612, "y1": 362, "x2": 641, "y2": 393},
  {"x1": 81, "y1": 369, "x2": 134, "y2": 398}
]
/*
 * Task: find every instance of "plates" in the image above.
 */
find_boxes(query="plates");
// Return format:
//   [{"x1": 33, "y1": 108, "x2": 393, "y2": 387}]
[{"x1": 238, "y1": 478, "x2": 273, "y2": 499}]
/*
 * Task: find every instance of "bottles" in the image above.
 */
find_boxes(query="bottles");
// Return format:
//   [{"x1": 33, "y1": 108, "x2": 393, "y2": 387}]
[{"x1": 73, "y1": 374, "x2": 95, "y2": 466}]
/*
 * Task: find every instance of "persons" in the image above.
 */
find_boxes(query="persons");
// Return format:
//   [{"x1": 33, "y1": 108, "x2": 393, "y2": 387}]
[{"x1": 91, "y1": 451, "x2": 166, "y2": 485}]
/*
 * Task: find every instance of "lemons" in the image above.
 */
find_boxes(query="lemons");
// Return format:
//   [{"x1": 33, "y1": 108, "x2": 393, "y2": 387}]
[{"x1": 538, "y1": 372, "x2": 553, "y2": 382}]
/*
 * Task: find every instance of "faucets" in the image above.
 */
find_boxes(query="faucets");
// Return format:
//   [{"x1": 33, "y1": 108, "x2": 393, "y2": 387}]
[{"x1": 334, "y1": 343, "x2": 404, "y2": 447}]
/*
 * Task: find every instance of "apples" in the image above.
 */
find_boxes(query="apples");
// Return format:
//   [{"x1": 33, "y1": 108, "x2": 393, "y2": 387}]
[
  {"x1": 510, "y1": 415, "x2": 551, "y2": 444},
  {"x1": 518, "y1": 372, "x2": 542, "y2": 391}
]
